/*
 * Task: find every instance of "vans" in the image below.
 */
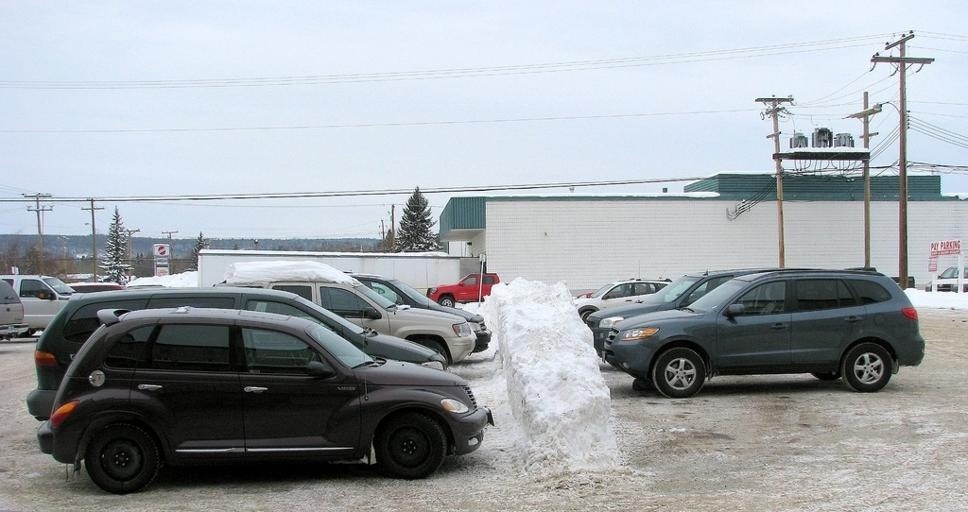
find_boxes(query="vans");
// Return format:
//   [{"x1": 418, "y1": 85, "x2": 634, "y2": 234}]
[{"x1": 0, "y1": 273, "x2": 122, "y2": 341}]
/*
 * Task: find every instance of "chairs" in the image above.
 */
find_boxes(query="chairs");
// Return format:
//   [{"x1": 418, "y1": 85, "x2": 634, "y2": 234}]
[{"x1": 761, "y1": 286, "x2": 778, "y2": 313}]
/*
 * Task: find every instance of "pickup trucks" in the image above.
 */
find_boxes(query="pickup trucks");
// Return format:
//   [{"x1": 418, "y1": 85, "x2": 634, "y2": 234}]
[{"x1": 426, "y1": 271, "x2": 509, "y2": 309}]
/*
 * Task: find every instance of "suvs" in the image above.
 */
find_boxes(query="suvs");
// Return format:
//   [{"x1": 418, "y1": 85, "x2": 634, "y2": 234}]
[
  {"x1": 584, "y1": 266, "x2": 787, "y2": 359},
  {"x1": 206, "y1": 260, "x2": 477, "y2": 367},
  {"x1": 23, "y1": 283, "x2": 449, "y2": 423},
  {"x1": 599, "y1": 266, "x2": 927, "y2": 400},
  {"x1": 573, "y1": 277, "x2": 696, "y2": 325}
]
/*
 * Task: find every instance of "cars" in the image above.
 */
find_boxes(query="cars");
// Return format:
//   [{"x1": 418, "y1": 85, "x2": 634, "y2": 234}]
[
  {"x1": 343, "y1": 270, "x2": 493, "y2": 353},
  {"x1": 35, "y1": 307, "x2": 497, "y2": 494},
  {"x1": 925, "y1": 265, "x2": 968, "y2": 292}
]
